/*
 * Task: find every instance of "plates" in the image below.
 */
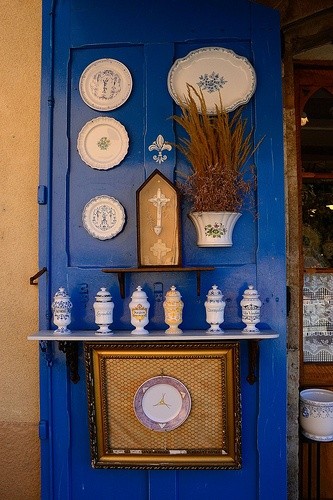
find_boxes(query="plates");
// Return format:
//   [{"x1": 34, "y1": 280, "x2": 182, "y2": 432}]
[
  {"x1": 79, "y1": 58, "x2": 133, "y2": 112},
  {"x1": 133, "y1": 376, "x2": 191, "y2": 431},
  {"x1": 167, "y1": 46, "x2": 257, "y2": 116},
  {"x1": 76, "y1": 116, "x2": 130, "y2": 171},
  {"x1": 82, "y1": 195, "x2": 126, "y2": 241}
]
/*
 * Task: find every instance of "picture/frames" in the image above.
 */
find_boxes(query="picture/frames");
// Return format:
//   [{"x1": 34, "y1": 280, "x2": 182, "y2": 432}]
[{"x1": 81, "y1": 342, "x2": 242, "y2": 471}]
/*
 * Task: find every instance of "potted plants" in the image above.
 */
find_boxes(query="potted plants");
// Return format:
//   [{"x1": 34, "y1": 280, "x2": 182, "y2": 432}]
[{"x1": 165, "y1": 83, "x2": 267, "y2": 248}]
